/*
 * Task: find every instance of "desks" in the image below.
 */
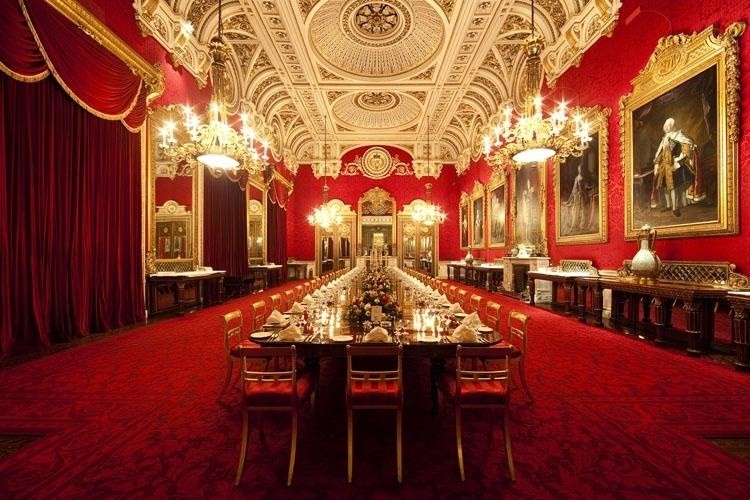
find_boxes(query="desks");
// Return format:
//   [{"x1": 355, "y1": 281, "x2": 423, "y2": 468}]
[
  {"x1": 146, "y1": 271, "x2": 228, "y2": 315},
  {"x1": 287, "y1": 261, "x2": 316, "y2": 279},
  {"x1": 527, "y1": 260, "x2": 750, "y2": 372}
]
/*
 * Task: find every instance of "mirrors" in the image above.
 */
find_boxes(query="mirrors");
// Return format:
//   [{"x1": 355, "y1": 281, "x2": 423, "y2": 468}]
[
  {"x1": 356, "y1": 187, "x2": 397, "y2": 257},
  {"x1": 246, "y1": 177, "x2": 267, "y2": 266},
  {"x1": 146, "y1": 102, "x2": 198, "y2": 263}
]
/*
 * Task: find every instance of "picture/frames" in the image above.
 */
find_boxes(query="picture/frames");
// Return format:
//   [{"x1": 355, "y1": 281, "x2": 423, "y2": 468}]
[
  {"x1": 618, "y1": 20, "x2": 746, "y2": 242},
  {"x1": 510, "y1": 160, "x2": 549, "y2": 257},
  {"x1": 459, "y1": 167, "x2": 510, "y2": 250},
  {"x1": 553, "y1": 104, "x2": 613, "y2": 245}
]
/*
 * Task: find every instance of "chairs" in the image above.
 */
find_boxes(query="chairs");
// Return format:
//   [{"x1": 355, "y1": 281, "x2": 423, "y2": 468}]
[
  {"x1": 341, "y1": 342, "x2": 408, "y2": 484},
  {"x1": 233, "y1": 342, "x2": 312, "y2": 486},
  {"x1": 214, "y1": 266, "x2": 532, "y2": 420},
  {"x1": 435, "y1": 339, "x2": 521, "y2": 483}
]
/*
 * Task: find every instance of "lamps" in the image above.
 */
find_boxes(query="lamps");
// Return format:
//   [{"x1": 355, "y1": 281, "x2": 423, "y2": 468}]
[
  {"x1": 481, "y1": 0, "x2": 593, "y2": 170},
  {"x1": 158, "y1": 0, "x2": 271, "y2": 181}
]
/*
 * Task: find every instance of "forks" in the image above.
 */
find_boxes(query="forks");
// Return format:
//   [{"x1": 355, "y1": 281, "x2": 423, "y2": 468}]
[{"x1": 355, "y1": 334, "x2": 362, "y2": 344}]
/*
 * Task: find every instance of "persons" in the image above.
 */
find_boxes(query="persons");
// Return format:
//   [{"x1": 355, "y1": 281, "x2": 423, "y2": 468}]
[
  {"x1": 562, "y1": 164, "x2": 595, "y2": 232},
  {"x1": 493, "y1": 198, "x2": 505, "y2": 241},
  {"x1": 522, "y1": 178, "x2": 534, "y2": 241},
  {"x1": 654, "y1": 118, "x2": 694, "y2": 217},
  {"x1": 475, "y1": 209, "x2": 481, "y2": 244},
  {"x1": 463, "y1": 215, "x2": 468, "y2": 246}
]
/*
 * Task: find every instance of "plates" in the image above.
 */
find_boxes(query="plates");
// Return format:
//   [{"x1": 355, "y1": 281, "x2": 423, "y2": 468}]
[
  {"x1": 250, "y1": 287, "x2": 331, "y2": 343},
  {"x1": 427, "y1": 291, "x2": 494, "y2": 343},
  {"x1": 418, "y1": 336, "x2": 438, "y2": 343},
  {"x1": 332, "y1": 335, "x2": 353, "y2": 342}
]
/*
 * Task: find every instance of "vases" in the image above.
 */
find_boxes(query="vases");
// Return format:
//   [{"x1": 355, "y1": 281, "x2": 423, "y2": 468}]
[
  {"x1": 632, "y1": 224, "x2": 662, "y2": 278},
  {"x1": 465, "y1": 245, "x2": 473, "y2": 267}
]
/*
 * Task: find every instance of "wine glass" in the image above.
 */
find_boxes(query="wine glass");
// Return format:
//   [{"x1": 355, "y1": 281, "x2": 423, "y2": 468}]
[{"x1": 393, "y1": 318, "x2": 411, "y2": 336}]
